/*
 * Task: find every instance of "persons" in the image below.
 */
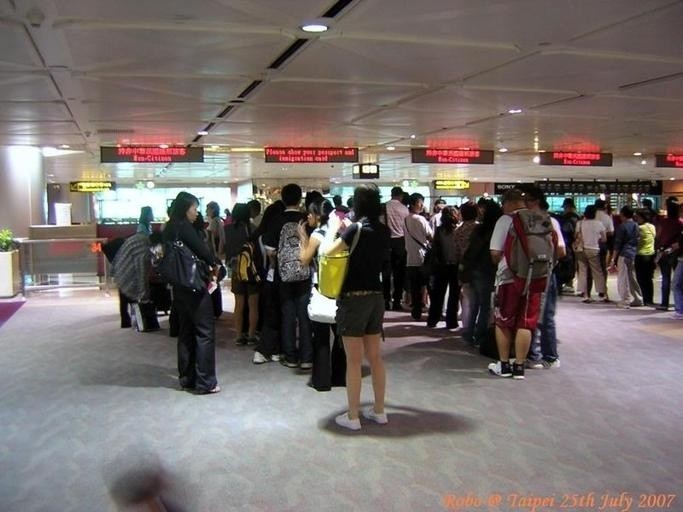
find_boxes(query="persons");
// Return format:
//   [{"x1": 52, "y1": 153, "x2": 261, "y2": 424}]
[
  {"x1": 524, "y1": 187, "x2": 566, "y2": 369},
  {"x1": 633, "y1": 208, "x2": 656, "y2": 306},
  {"x1": 319, "y1": 182, "x2": 392, "y2": 431},
  {"x1": 110, "y1": 229, "x2": 161, "y2": 332},
  {"x1": 454, "y1": 200, "x2": 479, "y2": 333},
  {"x1": 659, "y1": 202, "x2": 683, "y2": 320},
  {"x1": 344, "y1": 198, "x2": 356, "y2": 221},
  {"x1": 586, "y1": 199, "x2": 614, "y2": 296},
  {"x1": 402, "y1": 192, "x2": 409, "y2": 205},
  {"x1": 463, "y1": 201, "x2": 502, "y2": 348},
  {"x1": 427, "y1": 206, "x2": 461, "y2": 333},
  {"x1": 477, "y1": 196, "x2": 493, "y2": 225},
  {"x1": 248, "y1": 200, "x2": 263, "y2": 227},
  {"x1": 224, "y1": 209, "x2": 233, "y2": 225},
  {"x1": 429, "y1": 199, "x2": 447, "y2": 231},
  {"x1": 224, "y1": 203, "x2": 258, "y2": 346},
  {"x1": 333, "y1": 195, "x2": 349, "y2": 214},
  {"x1": 402, "y1": 285, "x2": 430, "y2": 312},
  {"x1": 262, "y1": 183, "x2": 313, "y2": 369},
  {"x1": 573, "y1": 205, "x2": 607, "y2": 303},
  {"x1": 610, "y1": 205, "x2": 643, "y2": 309},
  {"x1": 378, "y1": 186, "x2": 409, "y2": 312},
  {"x1": 161, "y1": 192, "x2": 220, "y2": 393},
  {"x1": 655, "y1": 196, "x2": 679, "y2": 309},
  {"x1": 561, "y1": 198, "x2": 582, "y2": 222},
  {"x1": 251, "y1": 203, "x2": 284, "y2": 363},
  {"x1": 642, "y1": 199, "x2": 657, "y2": 215},
  {"x1": 404, "y1": 193, "x2": 447, "y2": 321},
  {"x1": 137, "y1": 206, "x2": 153, "y2": 233},
  {"x1": 296, "y1": 197, "x2": 346, "y2": 392},
  {"x1": 488, "y1": 188, "x2": 547, "y2": 380},
  {"x1": 206, "y1": 201, "x2": 225, "y2": 259},
  {"x1": 193, "y1": 213, "x2": 222, "y2": 319}
]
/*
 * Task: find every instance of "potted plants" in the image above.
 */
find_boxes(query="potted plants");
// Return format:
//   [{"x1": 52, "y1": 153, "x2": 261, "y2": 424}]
[{"x1": 0, "y1": 229, "x2": 21, "y2": 298}]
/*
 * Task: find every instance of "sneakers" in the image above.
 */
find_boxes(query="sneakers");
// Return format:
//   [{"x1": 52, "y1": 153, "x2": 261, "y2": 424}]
[
  {"x1": 269, "y1": 353, "x2": 281, "y2": 361},
  {"x1": 300, "y1": 360, "x2": 314, "y2": 369},
  {"x1": 512, "y1": 361, "x2": 527, "y2": 380},
  {"x1": 281, "y1": 358, "x2": 300, "y2": 368},
  {"x1": 487, "y1": 361, "x2": 513, "y2": 378},
  {"x1": 360, "y1": 406, "x2": 389, "y2": 425},
  {"x1": 529, "y1": 355, "x2": 563, "y2": 371},
  {"x1": 251, "y1": 351, "x2": 265, "y2": 367},
  {"x1": 233, "y1": 336, "x2": 244, "y2": 348},
  {"x1": 245, "y1": 335, "x2": 261, "y2": 346},
  {"x1": 616, "y1": 300, "x2": 630, "y2": 308},
  {"x1": 579, "y1": 295, "x2": 589, "y2": 302},
  {"x1": 594, "y1": 295, "x2": 608, "y2": 302},
  {"x1": 334, "y1": 410, "x2": 362, "y2": 431},
  {"x1": 207, "y1": 385, "x2": 221, "y2": 392},
  {"x1": 666, "y1": 309, "x2": 683, "y2": 320}
]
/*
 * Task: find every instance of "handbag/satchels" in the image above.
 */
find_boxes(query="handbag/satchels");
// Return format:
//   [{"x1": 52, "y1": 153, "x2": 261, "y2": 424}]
[
  {"x1": 131, "y1": 298, "x2": 163, "y2": 333},
  {"x1": 305, "y1": 273, "x2": 337, "y2": 326},
  {"x1": 153, "y1": 223, "x2": 209, "y2": 295},
  {"x1": 403, "y1": 215, "x2": 440, "y2": 277},
  {"x1": 318, "y1": 221, "x2": 363, "y2": 300},
  {"x1": 571, "y1": 220, "x2": 585, "y2": 254},
  {"x1": 98, "y1": 231, "x2": 143, "y2": 263}
]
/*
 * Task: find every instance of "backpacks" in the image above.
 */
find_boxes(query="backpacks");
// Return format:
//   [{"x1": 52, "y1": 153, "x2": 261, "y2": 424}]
[
  {"x1": 233, "y1": 239, "x2": 265, "y2": 284},
  {"x1": 503, "y1": 208, "x2": 555, "y2": 296},
  {"x1": 276, "y1": 220, "x2": 311, "y2": 283}
]
